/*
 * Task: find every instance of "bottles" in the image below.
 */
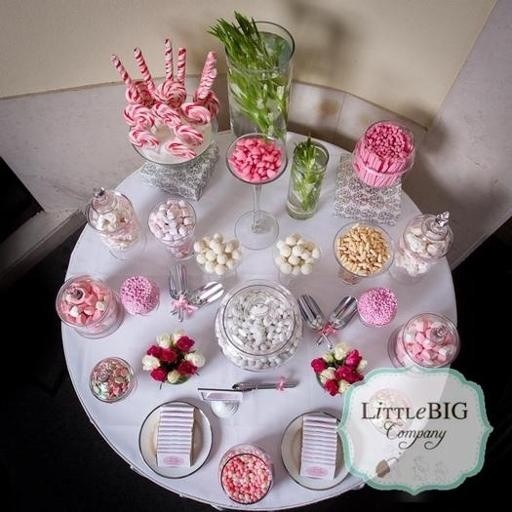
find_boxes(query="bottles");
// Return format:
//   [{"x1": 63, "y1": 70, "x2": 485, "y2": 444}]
[
  {"x1": 350, "y1": 119, "x2": 417, "y2": 190},
  {"x1": 85, "y1": 186, "x2": 145, "y2": 262},
  {"x1": 389, "y1": 211, "x2": 456, "y2": 285}
]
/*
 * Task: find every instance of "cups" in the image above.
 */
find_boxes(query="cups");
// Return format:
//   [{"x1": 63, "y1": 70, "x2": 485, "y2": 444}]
[
  {"x1": 129, "y1": 105, "x2": 218, "y2": 172},
  {"x1": 217, "y1": 444, "x2": 275, "y2": 505},
  {"x1": 55, "y1": 273, "x2": 124, "y2": 340},
  {"x1": 120, "y1": 276, "x2": 160, "y2": 317},
  {"x1": 356, "y1": 286, "x2": 399, "y2": 329},
  {"x1": 332, "y1": 219, "x2": 396, "y2": 286},
  {"x1": 224, "y1": 19, "x2": 296, "y2": 150},
  {"x1": 387, "y1": 311, "x2": 463, "y2": 372},
  {"x1": 146, "y1": 197, "x2": 197, "y2": 261}
]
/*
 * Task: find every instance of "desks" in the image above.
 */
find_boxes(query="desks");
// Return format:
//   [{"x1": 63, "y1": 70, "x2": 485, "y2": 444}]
[{"x1": 60, "y1": 125, "x2": 460, "y2": 512}]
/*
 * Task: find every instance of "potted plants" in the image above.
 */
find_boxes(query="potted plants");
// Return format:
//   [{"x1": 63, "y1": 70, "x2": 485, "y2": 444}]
[{"x1": 209, "y1": 15, "x2": 295, "y2": 137}]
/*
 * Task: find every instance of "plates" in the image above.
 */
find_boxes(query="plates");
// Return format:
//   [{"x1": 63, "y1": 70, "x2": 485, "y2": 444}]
[
  {"x1": 136, "y1": 400, "x2": 213, "y2": 480},
  {"x1": 279, "y1": 410, "x2": 354, "y2": 491}
]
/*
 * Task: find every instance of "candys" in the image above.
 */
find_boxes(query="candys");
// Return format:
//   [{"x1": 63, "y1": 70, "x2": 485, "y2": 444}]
[{"x1": 55, "y1": 38, "x2": 461, "y2": 506}]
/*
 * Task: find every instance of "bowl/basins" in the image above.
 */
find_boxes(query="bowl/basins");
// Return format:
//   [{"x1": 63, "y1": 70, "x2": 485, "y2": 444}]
[{"x1": 88, "y1": 355, "x2": 136, "y2": 404}]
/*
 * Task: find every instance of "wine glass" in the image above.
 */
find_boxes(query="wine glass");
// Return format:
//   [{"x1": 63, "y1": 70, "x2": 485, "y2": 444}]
[
  {"x1": 225, "y1": 132, "x2": 289, "y2": 252},
  {"x1": 271, "y1": 233, "x2": 322, "y2": 289},
  {"x1": 193, "y1": 230, "x2": 243, "y2": 288}
]
